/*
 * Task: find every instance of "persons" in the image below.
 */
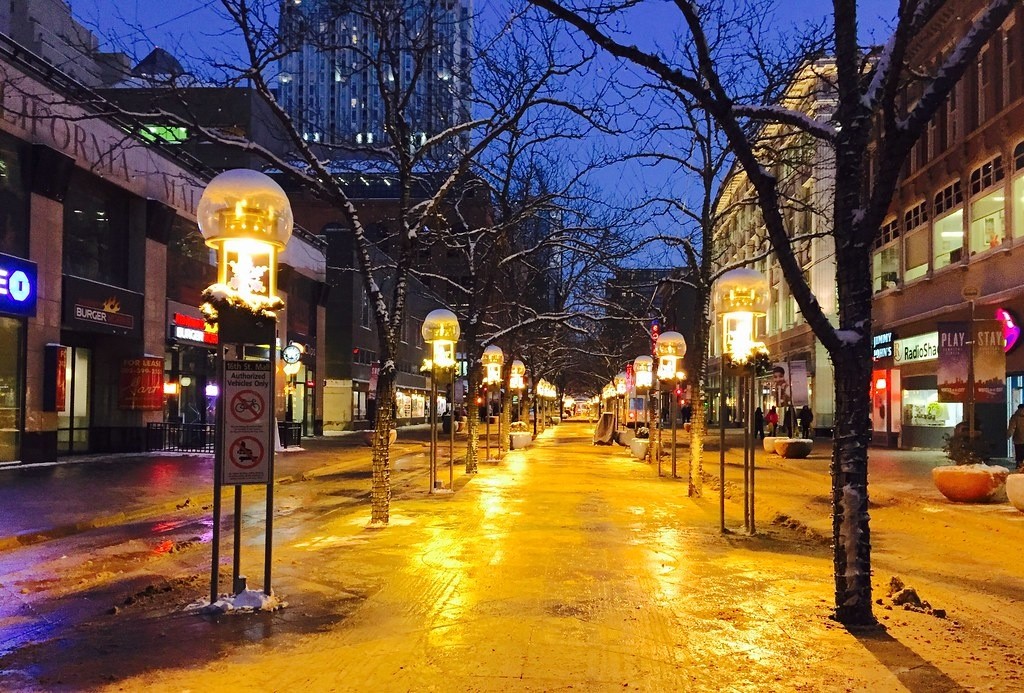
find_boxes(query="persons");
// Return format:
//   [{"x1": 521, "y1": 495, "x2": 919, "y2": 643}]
[
  {"x1": 680, "y1": 403, "x2": 687, "y2": 424},
  {"x1": 662, "y1": 403, "x2": 669, "y2": 423},
  {"x1": 455, "y1": 404, "x2": 499, "y2": 424},
  {"x1": 772, "y1": 366, "x2": 792, "y2": 406},
  {"x1": 798, "y1": 405, "x2": 813, "y2": 439},
  {"x1": 686, "y1": 403, "x2": 693, "y2": 422},
  {"x1": 765, "y1": 405, "x2": 779, "y2": 436},
  {"x1": 783, "y1": 406, "x2": 798, "y2": 438},
  {"x1": 1007, "y1": 403, "x2": 1024, "y2": 468},
  {"x1": 755, "y1": 406, "x2": 764, "y2": 440}
]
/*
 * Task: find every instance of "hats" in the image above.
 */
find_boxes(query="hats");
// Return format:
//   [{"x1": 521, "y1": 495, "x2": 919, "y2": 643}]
[
  {"x1": 772, "y1": 406, "x2": 776, "y2": 409},
  {"x1": 1018, "y1": 404, "x2": 1024, "y2": 408}
]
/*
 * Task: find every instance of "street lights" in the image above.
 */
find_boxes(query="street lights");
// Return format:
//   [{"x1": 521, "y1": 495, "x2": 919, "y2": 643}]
[
  {"x1": 195, "y1": 169, "x2": 294, "y2": 606},
  {"x1": 712, "y1": 269, "x2": 772, "y2": 537},
  {"x1": 509, "y1": 359, "x2": 526, "y2": 423},
  {"x1": 633, "y1": 355, "x2": 653, "y2": 437},
  {"x1": 481, "y1": 345, "x2": 505, "y2": 460},
  {"x1": 602, "y1": 372, "x2": 627, "y2": 431},
  {"x1": 655, "y1": 331, "x2": 688, "y2": 478},
  {"x1": 421, "y1": 308, "x2": 461, "y2": 494},
  {"x1": 536, "y1": 378, "x2": 557, "y2": 433}
]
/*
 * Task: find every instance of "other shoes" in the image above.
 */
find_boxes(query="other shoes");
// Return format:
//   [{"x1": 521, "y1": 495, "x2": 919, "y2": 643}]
[{"x1": 1016, "y1": 465, "x2": 1020, "y2": 468}]
